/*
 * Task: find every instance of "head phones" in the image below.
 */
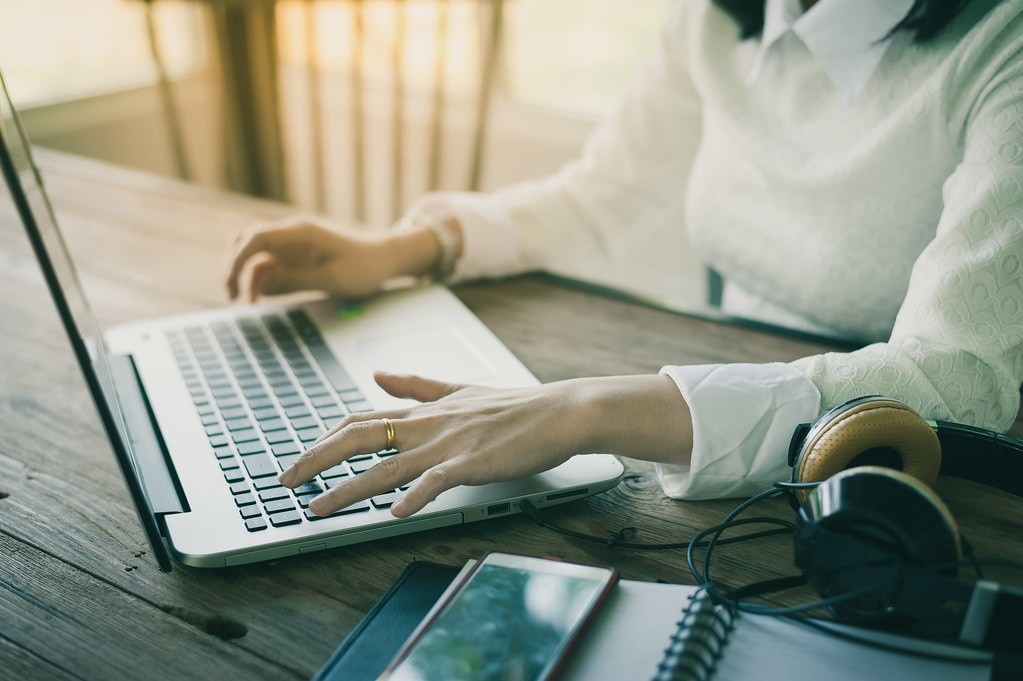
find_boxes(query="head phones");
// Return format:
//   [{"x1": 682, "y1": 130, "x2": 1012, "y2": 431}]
[{"x1": 788, "y1": 395, "x2": 1023, "y2": 649}]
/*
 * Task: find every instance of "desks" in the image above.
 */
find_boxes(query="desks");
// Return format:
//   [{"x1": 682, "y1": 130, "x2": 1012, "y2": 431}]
[{"x1": 1, "y1": 140, "x2": 1023, "y2": 681}]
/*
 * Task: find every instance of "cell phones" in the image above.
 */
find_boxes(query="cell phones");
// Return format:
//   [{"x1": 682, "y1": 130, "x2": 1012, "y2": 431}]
[{"x1": 382, "y1": 549, "x2": 621, "y2": 681}]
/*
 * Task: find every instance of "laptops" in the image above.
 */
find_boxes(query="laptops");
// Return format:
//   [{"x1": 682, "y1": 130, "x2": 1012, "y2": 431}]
[{"x1": 0, "y1": 71, "x2": 626, "y2": 573}]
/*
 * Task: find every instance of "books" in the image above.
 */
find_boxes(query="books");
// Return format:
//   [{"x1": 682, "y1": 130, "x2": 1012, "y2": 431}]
[{"x1": 314, "y1": 558, "x2": 995, "y2": 681}]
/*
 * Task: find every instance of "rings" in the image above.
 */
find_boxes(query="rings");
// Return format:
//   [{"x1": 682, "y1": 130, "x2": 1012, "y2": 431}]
[{"x1": 381, "y1": 418, "x2": 398, "y2": 451}]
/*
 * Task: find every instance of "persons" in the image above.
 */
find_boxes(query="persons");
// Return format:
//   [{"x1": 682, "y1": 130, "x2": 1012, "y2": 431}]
[{"x1": 223, "y1": 0, "x2": 1023, "y2": 523}]
